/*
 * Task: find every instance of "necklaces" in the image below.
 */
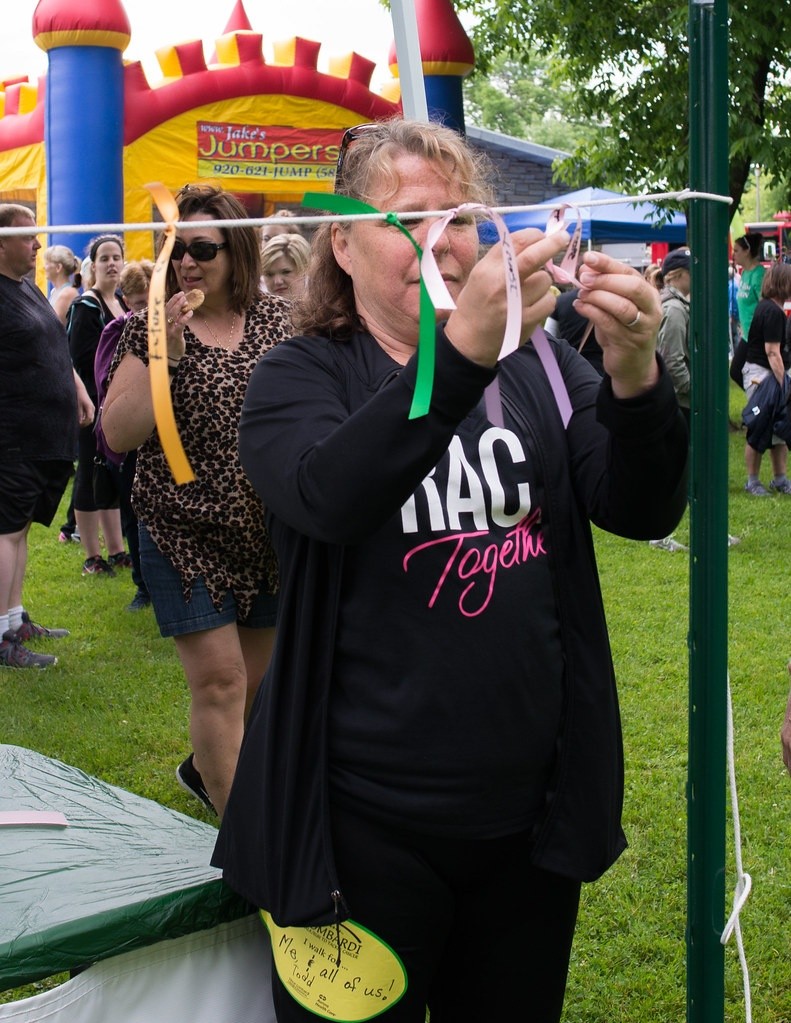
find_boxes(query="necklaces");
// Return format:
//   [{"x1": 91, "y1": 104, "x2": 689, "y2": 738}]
[{"x1": 200, "y1": 309, "x2": 235, "y2": 349}]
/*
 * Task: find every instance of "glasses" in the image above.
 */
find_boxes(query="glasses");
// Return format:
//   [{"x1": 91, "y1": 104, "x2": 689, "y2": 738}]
[
  {"x1": 170, "y1": 242, "x2": 227, "y2": 262},
  {"x1": 334, "y1": 123, "x2": 391, "y2": 194}
]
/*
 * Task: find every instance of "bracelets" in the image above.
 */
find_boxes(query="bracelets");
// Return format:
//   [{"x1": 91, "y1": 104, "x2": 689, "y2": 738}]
[
  {"x1": 167, "y1": 355, "x2": 180, "y2": 362},
  {"x1": 168, "y1": 366, "x2": 178, "y2": 375}
]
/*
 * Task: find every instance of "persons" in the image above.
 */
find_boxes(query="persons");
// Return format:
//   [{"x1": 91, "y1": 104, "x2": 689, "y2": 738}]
[
  {"x1": 209, "y1": 122, "x2": 690, "y2": 1023},
  {"x1": 100, "y1": 182, "x2": 305, "y2": 822},
  {"x1": 0, "y1": 203, "x2": 791, "y2": 670}
]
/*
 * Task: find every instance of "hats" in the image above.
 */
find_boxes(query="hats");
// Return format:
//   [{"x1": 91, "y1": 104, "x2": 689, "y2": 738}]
[{"x1": 661, "y1": 250, "x2": 691, "y2": 275}]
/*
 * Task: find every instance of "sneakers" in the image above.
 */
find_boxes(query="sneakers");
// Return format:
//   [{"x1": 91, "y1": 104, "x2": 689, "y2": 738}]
[
  {"x1": 727, "y1": 534, "x2": 740, "y2": 547},
  {"x1": 176, "y1": 753, "x2": 219, "y2": 817},
  {"x1": 745, "y1": 480, "x2": 772, "y2": 497},
  {"x1": 0, "y1": 630, "x2": 58, "y2": 670},
  {"x1": 649, "y1": 532, "x2": 689, "y2": 553},
  {"x1": 108, "y1": 553, "x2": 132, "y2": 568},
  {"x1": 81, "y1": 556, "x2": 116, "y2": 579},
  {"x1": 769, "y1": 479, "x2": 791, "y2": 495},
  {"x1": 14, "y1": 612, "x2": 71, "y2": 641}
]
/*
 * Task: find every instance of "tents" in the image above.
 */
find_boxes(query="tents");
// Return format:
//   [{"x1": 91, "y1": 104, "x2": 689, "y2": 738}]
[{"x1": 476, "y1": 186, "x2": 689, "y2": 253}]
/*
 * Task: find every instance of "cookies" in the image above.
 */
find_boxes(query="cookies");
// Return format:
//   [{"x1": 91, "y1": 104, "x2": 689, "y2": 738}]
[{"x1": 181, "y1": 289, "x2": 205, "y2": 313}]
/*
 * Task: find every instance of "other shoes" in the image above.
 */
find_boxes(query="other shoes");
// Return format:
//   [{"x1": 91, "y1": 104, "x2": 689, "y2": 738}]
[
  {"x1": 59, "y1": 532, "x2": 80, "y2": 542},
  {"x1": 730, "y1": 423, "x2": 739, "y2": 432},
  {"x1": 128, "y1": 590, "x2": 150, "y2": 611},
  {"x1": 741, "y1": 423, "x2": 748, "y2": 429}
]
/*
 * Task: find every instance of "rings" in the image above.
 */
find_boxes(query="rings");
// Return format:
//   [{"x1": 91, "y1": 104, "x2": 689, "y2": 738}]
[
  {"x1": 624, "y1": 309, "x2": 640, "y2": 327},
  {"x1": 167, "y1": 316, "x2": 177, "y2": 325}
]
